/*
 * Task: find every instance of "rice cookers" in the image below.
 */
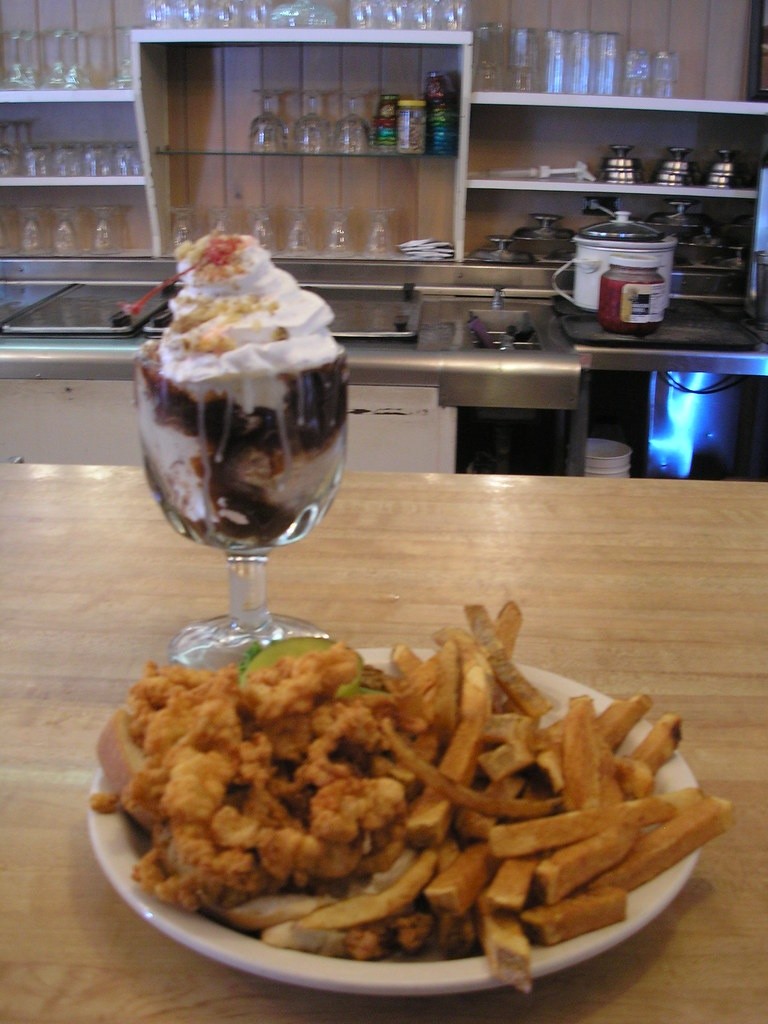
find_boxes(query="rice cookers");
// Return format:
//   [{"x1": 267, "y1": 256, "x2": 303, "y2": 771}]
[{"x1": 573, "y1": 210, "x2": 675, "y2": 311}]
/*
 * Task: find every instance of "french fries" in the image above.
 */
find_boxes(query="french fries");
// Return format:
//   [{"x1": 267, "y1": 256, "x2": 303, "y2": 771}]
[{"x1": 259, "y1": 600, "x2": 731, "y2": 995}]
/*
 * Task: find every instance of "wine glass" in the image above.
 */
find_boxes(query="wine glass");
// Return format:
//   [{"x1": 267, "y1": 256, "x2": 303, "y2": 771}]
[
  {"x1": 51, "y1": 207, "x2": 79, "y2": 252},
  {"x1": 132, "y1": 345, "x2": 350, "y2": 676},
  {"x1": 322, "y1": 206, "x2": 356, "y2": 257},
  {"x1": 250, "y1": 90, "x2": 289, "y2": 149},
  {"x1": 0, "y1": 120, "x2": 27, "y2": 176},
  {"x1": 284, "y1": 205, "x2": 315, "y2": 256},
  {"x1": 295, "y1": 90, "x2": 336, "y2": 150},
  {"x1": 0, "y1": 26, "x2": 134, "y2": 90},
  {"x1": 170, "y1": 206, "x2": 198, "y2": 250},
  {"x1": 337, "y1": 94, "x2": 370, "y2": 152},
  {"x1": 210, "y1": 208, "x2": 234, "y2": 235},
  {"x1": 246, "y1": 205, "x2": 278, "y2": 255},
  {"x1": 19, "y1": 207, "x2": 46, "y2": 255},
  {"x1": 88, "y1": 206, "x2": 122, "y2": 255},
  {"x1": 363, "y1": 208, "x2": 395, "y2": 258}
]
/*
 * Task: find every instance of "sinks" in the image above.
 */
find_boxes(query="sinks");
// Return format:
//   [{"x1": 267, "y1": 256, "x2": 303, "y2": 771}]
[{"x1": 465, "y1": 308, "x2": 544, "y2": 351}]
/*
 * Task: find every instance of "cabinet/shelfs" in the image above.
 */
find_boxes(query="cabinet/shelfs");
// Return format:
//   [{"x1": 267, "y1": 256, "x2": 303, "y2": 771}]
[
  {"x1": 0, "y1": 28, "x2": 768, "y2": 264},
  {"x1": 0, "y1": 377, "x2": 456, "y2": 472}
]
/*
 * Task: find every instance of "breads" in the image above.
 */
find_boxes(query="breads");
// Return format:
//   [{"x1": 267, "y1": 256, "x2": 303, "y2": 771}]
[{"x1": 96, "y1": 707, "x2": 418, "y2": 930}]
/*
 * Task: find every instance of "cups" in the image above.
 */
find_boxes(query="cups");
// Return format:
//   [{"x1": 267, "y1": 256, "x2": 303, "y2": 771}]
[
  {"x1": 508, "y1": 28, "x2": 541, "y2": 92},
  {"x1": 570, "y1": 30, "x2": 595, "y2": 93},
  {"x1": 23, "y1": 139, "x2": 141, "y2": 176},
  {"x1": 652, "y1": 51, "x2": 676, "y2": 98},
  {"x1": 473, "y1": 22, "x2": 507, "y2": 90},
  {"x1": 595, "y1": 31, "x2": 624, "y2": 95},
  {"x1": 543, "y1": 29, "x2": 569, "y2": 94},
  {"x1": 626, "y1": 49, "x2": 651, "y2": 97}
]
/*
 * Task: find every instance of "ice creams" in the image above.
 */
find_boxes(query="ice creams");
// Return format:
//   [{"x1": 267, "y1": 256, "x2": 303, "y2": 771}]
[{"x1": 132, "y1": 232, "x2": 349, "y2": 552}]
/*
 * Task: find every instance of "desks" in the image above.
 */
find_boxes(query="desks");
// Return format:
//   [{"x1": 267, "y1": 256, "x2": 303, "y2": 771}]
[{"x1": 0, "y1": 462, "x2": 768, "y2": 1024}]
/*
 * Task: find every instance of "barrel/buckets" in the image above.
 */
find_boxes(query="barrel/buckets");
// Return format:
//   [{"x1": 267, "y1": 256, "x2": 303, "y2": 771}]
[{"x1": 584, "y1": 438, "x2": 633, "y2": 477}]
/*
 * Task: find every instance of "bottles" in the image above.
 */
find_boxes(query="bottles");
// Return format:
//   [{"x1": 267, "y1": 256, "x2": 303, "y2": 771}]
[{"x1": 469, "y1": 316, "x2": 495, "y2": 347}]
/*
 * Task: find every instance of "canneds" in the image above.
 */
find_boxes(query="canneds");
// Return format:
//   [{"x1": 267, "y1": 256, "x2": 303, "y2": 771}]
[
  {"x1": 395, "y1": 99, "x2": 427, "y2": 154},
  {"x1": 597, "y1": 253, "x2": 665, "y2": 336}
]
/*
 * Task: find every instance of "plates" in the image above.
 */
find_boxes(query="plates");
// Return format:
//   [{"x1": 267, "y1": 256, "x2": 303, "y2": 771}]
[{"x1": 88, "y1": 650, "x2": 702, "y2": 993}]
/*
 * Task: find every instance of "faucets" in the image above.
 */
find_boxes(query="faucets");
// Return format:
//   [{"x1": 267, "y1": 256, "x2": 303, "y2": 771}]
[{"x1": 492, "y1": 284, "x2": 506, "y2": 312}]
